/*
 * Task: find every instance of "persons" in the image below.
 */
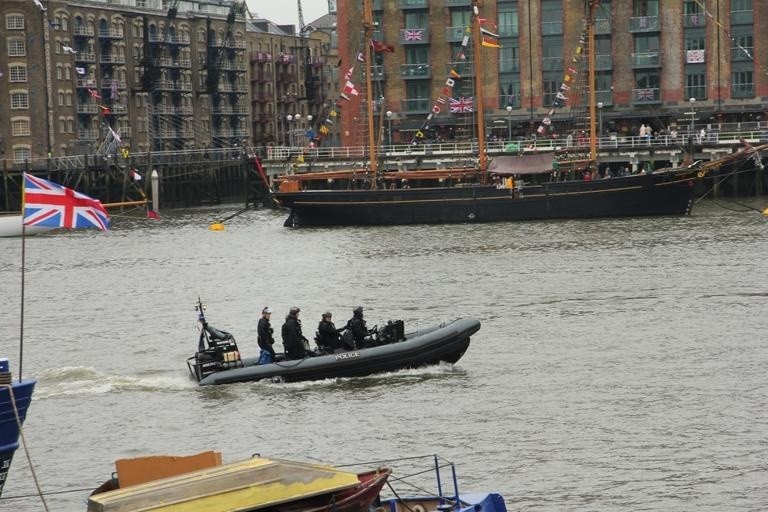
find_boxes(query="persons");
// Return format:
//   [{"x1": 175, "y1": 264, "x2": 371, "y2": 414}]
[
  {"x1": 282, "y1": 306, "x2": 305, "y2": 359},
  {"x1": 319, "y1": 312, "x2": 347, "y2": 350},
  {"x1": 565, "y1": 166, "x2": 630, "y2": 181},
  {"x1": 493, "y1": 176, "x2": 524, "y2": 190},
  {"x1": 258, "y1": 306, "x2": 277, "y2": 358},
  {"x1": 347, "y1": 307, "x2": 369, "y2": 349},
  {"x1": 378, "y1": 176, "x2": 410, "y2": 189}
]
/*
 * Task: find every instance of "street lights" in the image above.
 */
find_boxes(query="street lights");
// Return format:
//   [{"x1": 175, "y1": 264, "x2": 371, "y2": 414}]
[
  {"x1": 307, "y1": 114, "x2": 313, "y2": 148},
  {"x1": 597, "y1": 102, "x2": 603, "y2": 146},
  {"x1": 386, "y1": 110, "x2": 394, "y2": 157},
  {"x1": 689, "y1": 97, "x2": 696, "y2": 139},
  {"x1": 285, "y1": 114, "x2": 293, "y2": 146},
  {"x1": 294, "y1": 113, "x2": 302, "y2": 147},
  {"x1": 506, "y1": 105, "x2": 513, "y2": 143}
]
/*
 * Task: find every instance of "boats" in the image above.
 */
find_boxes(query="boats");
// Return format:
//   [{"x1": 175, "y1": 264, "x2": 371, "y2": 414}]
[
  {"x1": 0, "y1": 356, "x2": 36, "y2": 497},
  {"x1": 186, "y1": 295, "x2": 481, "y2": 386},
  {"x1": 90, "y1": 466, "x2": 393, "y2": 511},
  {"x1": 88, "y1": 454, "x2": 505, "y2": 512}
]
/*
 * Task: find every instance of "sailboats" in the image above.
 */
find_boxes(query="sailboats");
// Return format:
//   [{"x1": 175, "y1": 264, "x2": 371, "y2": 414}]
[{"x1": 267, "y1": 1, "x2": 768, "y2": 227}]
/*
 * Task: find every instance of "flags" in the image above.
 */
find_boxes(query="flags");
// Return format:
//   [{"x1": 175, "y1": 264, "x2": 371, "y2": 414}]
[
  {"x1": 148, "y1": 211, "x2": 159, "y2": 219},
  {"x1": 403, "y1": 16, "x2": 503, "y2": 154},
  {"x1": 288, "y1": 14, "x2": 394, "y2": 176},
  {"x1": 686, "y1": 49, "x2": 703, "y2": 63},
  {"x1": 34, "y1": 0, "x2": 145, "y2": 183},
  {"x1": 635, "y1": 88, "x2": 654, "y2": 102},
  {"x1": 527, "y1": 15, "x2": 592, "y2": 149},
  {"x1": 20, "y1": 173, "x2": 112, "y2": 233}
]
